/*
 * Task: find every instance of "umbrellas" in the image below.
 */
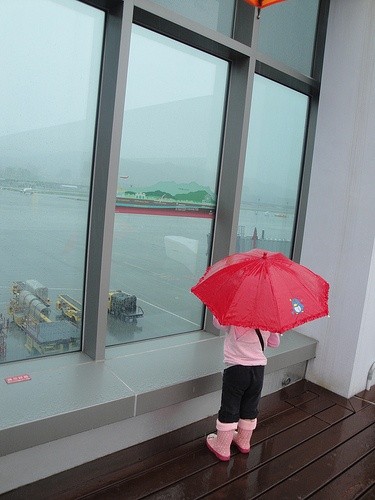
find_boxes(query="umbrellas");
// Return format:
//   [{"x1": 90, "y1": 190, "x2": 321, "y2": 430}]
[{"x1": 191, "y1": 248, "x2": 331, "y2": 336}]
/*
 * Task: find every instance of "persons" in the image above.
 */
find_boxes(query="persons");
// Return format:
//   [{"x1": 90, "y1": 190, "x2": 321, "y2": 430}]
[{"x1": 207, "y1": 314, "x2": 280, "y2": 461}]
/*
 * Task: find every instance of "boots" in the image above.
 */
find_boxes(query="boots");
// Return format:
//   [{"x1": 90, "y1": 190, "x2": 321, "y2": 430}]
[
  {"x1": 205, "y1": 419, "x2": 238, "y2": 461},
  {"x1": 232, "y1": 417, "x2": 257, "y2": 453}
]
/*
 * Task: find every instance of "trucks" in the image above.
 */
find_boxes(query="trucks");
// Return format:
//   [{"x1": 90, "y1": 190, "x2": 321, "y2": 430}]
[{"x1": 109, "y1": 291, "x2": 144, "y2": 323}]
